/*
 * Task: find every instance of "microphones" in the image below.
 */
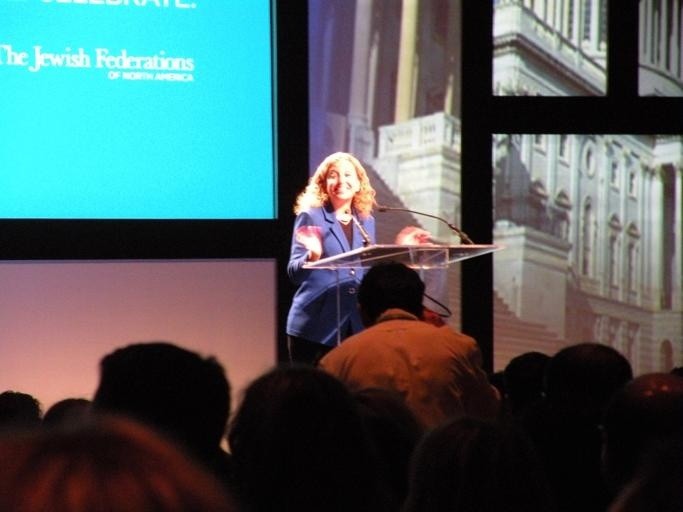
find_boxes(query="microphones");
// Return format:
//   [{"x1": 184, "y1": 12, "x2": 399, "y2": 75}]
[
  {"x1": 378, "y1": 206, "x2": 474, "y2": 244},
  {"x1": 345, "y1": 208, "x2": 370, "y2": 247}
]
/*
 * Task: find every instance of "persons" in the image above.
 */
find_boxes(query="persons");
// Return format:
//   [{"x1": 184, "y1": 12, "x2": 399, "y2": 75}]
[
  {"x1": 1, "y1": 259, "x2": 682, "y2": 512},
  {"x1": 284, "y1": 152, "x2": 432, "y2": 366}
]
